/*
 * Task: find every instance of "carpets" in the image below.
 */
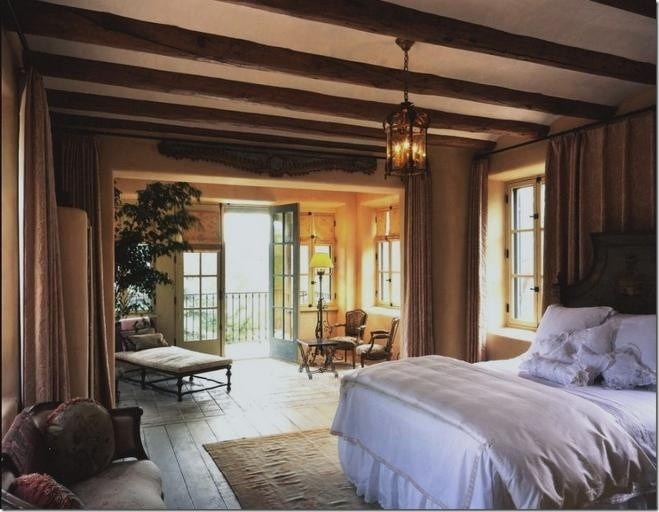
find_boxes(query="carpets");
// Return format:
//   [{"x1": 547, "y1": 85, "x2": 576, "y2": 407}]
[{"x1": 202, "y1": 428, "x2": 384, "y2": 510}]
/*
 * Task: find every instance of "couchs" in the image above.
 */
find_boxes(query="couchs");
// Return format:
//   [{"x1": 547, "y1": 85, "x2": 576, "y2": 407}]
[{"x1": 1, "y1": 402, "x2": 165, "y2": 511}]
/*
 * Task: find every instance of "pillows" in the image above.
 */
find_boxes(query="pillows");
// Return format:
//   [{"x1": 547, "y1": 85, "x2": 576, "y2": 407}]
[
  {"x1": 605, "y1": 314, "x2": 656, "y2": 369},
  {"x1": 120, "y1": 328, "x2": 156, "y2": 351},
  {"x1": 47, "y1": 398, "x2": 115, "y2": 477},
  {"x1": 9, "y1": 474, "x2": 86, "y2": 509},
  {"x1": 529, "y1": 304, "x2": 610, "y2": 350},
  {"x1": 128, "y1": 333, "x2": 169, "y2": 350},
  {"x1": 570, "y1": 321, "x2": 612, "y2": 354},
  {"x1": 601, "y1": 346, "x2": 656, "y2": 389}
]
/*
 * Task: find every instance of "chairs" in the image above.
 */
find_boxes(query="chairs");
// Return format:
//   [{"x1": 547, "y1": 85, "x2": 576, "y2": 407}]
[
  {"x1": 356, "y1": 317, "x2": 400, "y2": 367},
  {"x1": 331, "y1": 309, "x2": 368, "y2": 362}
]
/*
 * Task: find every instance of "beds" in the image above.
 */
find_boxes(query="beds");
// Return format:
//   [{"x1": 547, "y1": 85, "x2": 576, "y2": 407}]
[
  {"x1": 331, "y1": 231, "x2": 658, "y2": 510},
  {"x1": 115, "y1": 316, "x2": 233, "y2": 402}
]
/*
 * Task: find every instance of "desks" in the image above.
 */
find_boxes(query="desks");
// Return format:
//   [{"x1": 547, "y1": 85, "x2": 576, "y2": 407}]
[{"x1": 297, "y1": 339, "x2": 338, "y2": 380}]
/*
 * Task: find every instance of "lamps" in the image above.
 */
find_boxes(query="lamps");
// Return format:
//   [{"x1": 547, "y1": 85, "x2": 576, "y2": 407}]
[
  {"x1": 384, "y1": 38, "x2": 430, "y2": 180},
  {"x1": 310, "y1": 252, "x2": 334, "y2": 338}
]
